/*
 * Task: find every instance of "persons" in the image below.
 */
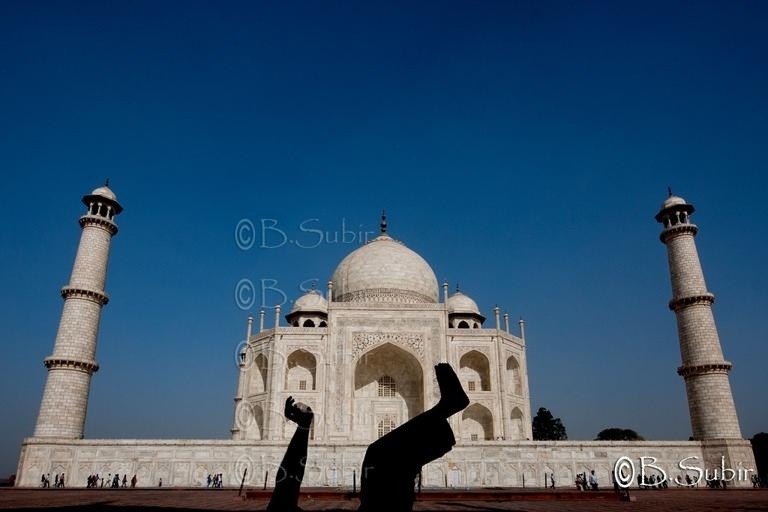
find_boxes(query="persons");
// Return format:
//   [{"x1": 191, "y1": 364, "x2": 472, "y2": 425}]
[
  {"x1": 550, "y1": 470, "x2": 762, "y2": 501},
  {"x1": 9, "y1": 473, "x2": 223, "y2": 488},
  {"x1": 266, "y1": 362, "x2": 470, "y2": 512}
]
